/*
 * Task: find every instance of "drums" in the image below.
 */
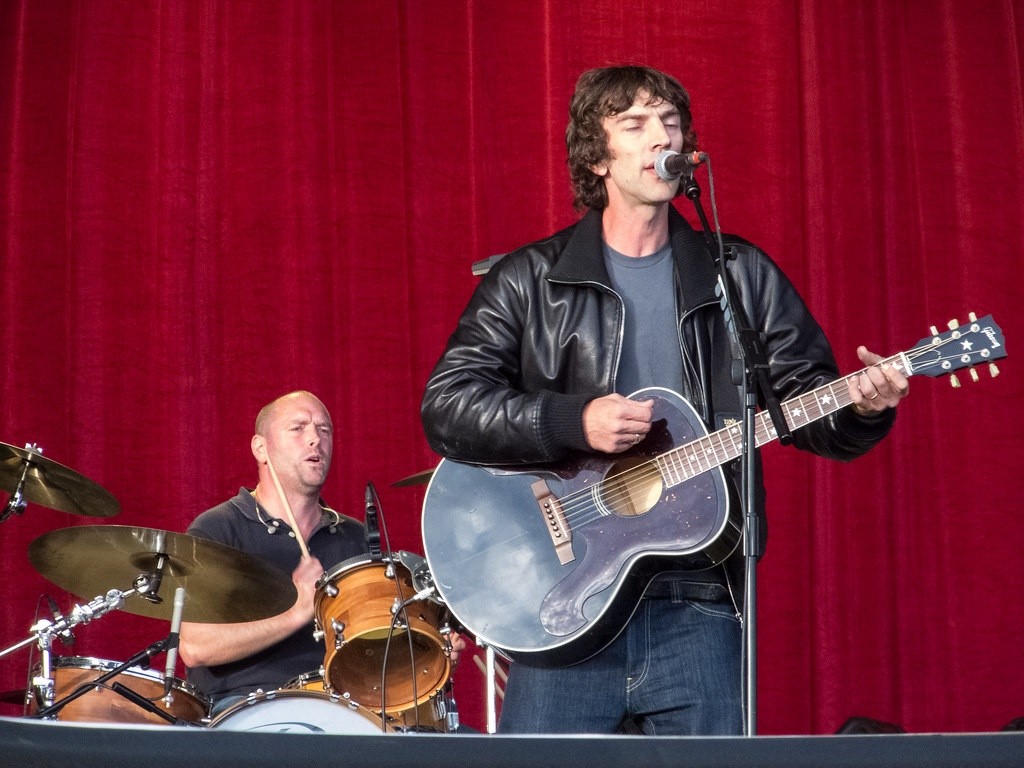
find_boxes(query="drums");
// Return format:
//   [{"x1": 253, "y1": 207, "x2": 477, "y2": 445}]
[
  {"x1": 202, "y1": 686, "x2": 398, "y2": 736},
  {"x1": 26, "y1": 654, "x2": 214, "y2": 733},
  {"x1": 314, "y1": 550, "x2": 452, "y2": 712},
  {"x1": 283, "y1": 667, "x2": 450, "y2": 731}
]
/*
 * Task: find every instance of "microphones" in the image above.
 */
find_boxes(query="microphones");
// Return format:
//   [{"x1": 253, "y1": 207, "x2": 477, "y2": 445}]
[
  {"x1": 365, "y1": 484, "x2": 374, "y2": 543},
  {"x1": 47, "y1": 597, "x2": 75, "y2": 644},
  {"x1": 654, "y1": 150, "x2": 708, "y2": 182},
  {"x1": 163, "y1": 587, "x2": 185, "y2": 680}
]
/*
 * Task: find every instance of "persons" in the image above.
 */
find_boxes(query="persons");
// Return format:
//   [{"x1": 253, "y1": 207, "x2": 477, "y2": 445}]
[
  {"x1": 420, "y1": 56, "x2": 911, "y2": 736},
  {"x1": 180, "y1": 392, "x2": 483, "y2": 734}
]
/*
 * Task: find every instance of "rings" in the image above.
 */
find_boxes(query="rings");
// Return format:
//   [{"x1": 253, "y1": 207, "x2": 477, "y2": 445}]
[
  {"x1": 629, "y1": 434, "x2": 641, "y2": 445},
  {"x1": 866, "y1": 391, "x2": 880, "y2": 400}
]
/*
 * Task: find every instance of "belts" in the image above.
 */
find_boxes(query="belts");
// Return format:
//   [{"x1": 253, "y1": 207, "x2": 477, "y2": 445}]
[{"x1": 641, "y1": 579, "x2": 735, "y2": 602}]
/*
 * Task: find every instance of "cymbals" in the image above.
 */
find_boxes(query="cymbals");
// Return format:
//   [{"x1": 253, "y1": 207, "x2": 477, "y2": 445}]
[
  {"x1": 0, "y1": 441, "x2": 122, "y2": 518},
  {"x1": 27, "y1": 523, "x2": 299, "y2": 625}
]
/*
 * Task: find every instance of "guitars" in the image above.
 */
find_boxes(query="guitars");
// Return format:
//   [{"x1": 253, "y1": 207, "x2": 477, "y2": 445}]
[{"x1": 420, "y1": 310, "x2": 1009, "y2": 671}]
